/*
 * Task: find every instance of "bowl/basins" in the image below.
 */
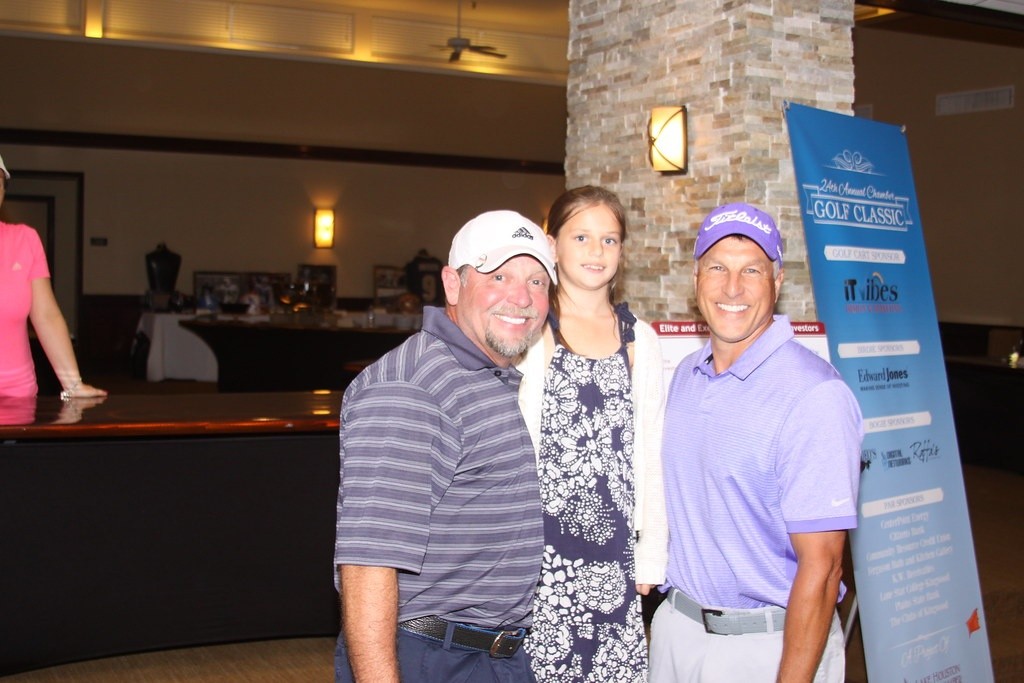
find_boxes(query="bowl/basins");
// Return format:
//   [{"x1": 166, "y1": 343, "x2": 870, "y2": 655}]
[{"x1": 395, "y1": 317, "x2": 414, "y2": 330}]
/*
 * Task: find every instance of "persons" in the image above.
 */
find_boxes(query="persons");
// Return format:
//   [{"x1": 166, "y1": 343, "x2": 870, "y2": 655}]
[
  {"x1": 519, "y1": 184, "x2": 668, "y2": 681},
  {"x1": 648, "y1": 201, "x2": 866, "y2": 683},
  {"x1": 0, "y1": 151, "x2": 109, "y2": 399},
  {"x1": 330, "y1": 207, "x2": 558, "y2": 682}
]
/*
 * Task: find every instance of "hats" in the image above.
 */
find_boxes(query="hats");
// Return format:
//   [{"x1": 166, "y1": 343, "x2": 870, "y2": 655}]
[
  {"x1": 0, "y1": 153, "x2": 9, "y2": 178},
  {"x1": 447, "y1": 209, "x2": 558, "y2": 285},
  {"x1": 693, "y1": 202, "x2": 784, "y2": 270}
]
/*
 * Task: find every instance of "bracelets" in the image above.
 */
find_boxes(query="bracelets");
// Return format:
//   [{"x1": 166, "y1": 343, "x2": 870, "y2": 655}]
[{"x1": 60, "y1": 377, "x2": 83, "y2": 404}]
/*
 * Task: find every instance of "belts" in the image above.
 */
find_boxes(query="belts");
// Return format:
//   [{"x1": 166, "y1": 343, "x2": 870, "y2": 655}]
[
  {"x1": 666, "y1": 587, "x2": 785, "y2": 634},
  {"x1": 399, "y1": 614, "x2": 527, "y2": 659}
]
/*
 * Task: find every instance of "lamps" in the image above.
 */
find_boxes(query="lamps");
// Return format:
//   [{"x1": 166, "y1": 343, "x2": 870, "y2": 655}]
[
  {"x1": 313, "y1": 207, "x2": 335, "y2": 249},
  {"x1": 647, "y1": 104, "x2": 689, "y2": 174}
]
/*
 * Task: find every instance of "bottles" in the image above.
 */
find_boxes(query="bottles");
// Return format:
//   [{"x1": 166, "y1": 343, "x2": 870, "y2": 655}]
[{"x1": 367, "y1": 306, "x2": 375, "y2": 328}]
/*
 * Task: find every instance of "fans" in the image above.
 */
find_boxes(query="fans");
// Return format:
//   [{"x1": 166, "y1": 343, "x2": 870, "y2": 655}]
[{"x1": 430, "y1": 0, "x2": 508, "y2": 60}]
